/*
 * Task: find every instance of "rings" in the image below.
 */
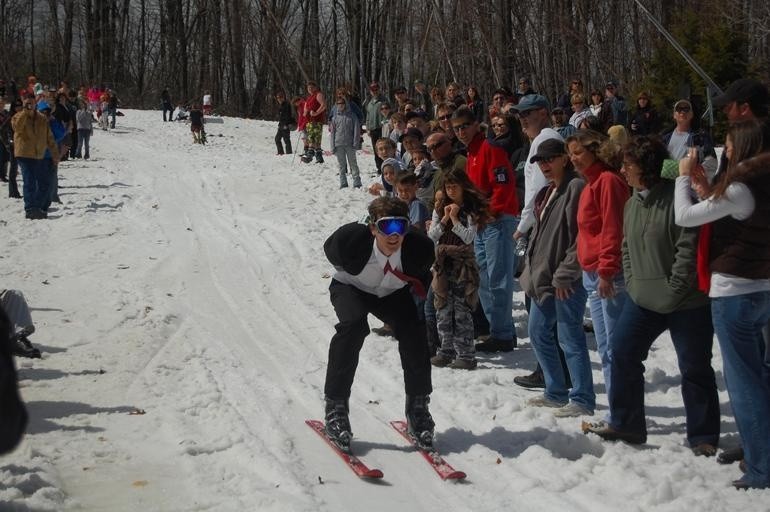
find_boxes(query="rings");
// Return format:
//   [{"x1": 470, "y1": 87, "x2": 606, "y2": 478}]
[{"x1": 687, "y1": 153, "x2": 690, "y2": 157}]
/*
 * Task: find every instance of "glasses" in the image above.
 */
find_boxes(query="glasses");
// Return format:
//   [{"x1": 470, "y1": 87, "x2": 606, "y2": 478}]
[
  {"x1": 676, "y1": 107, "x2": 689, "y2": 112},
  {"x1": 376, "y1": 215, "x2": 410, "y2": 237},
  {"x1": 380, "y1": 90, "x2": 564, "y2": 164}
]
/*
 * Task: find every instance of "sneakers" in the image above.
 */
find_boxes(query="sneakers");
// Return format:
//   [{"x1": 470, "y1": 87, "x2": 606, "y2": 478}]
[
  {"x1": 325, "y1": 398, "x2": 353, "y2": 452},
  {"x1": 10, "y1": 334, "x2": 40, "y2": 358},
  {"x1": 555, "y1": 403, "x2": 594, "y2": 418},
  {"x1": 405, "y1": 393, "x2": 436, "y2": 447},
  {"x1": 525, "y1": 395, "x2": 569, "y2": 408}
]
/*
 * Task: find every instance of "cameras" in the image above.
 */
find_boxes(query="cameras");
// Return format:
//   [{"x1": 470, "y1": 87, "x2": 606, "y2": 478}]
[{"x1": 688, "y1": 129, "x2": 706, "y2": 148}]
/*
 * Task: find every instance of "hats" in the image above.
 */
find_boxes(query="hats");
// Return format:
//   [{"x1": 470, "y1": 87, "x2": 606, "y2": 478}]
[
  {"x1": 393, "y1": 87, "x2": 406, "y2": 91},
  {"x1": 369, "y1": 83, "x2": 379, "y2": 87},
  {"x1": 606, "y1": 82, "x2": 617, "y2": 89},
  {"x1": 413, "y1": 79, "x2": 423, "y2": 84},
  {"x1": 674, "y1": 100, "x2": 693, "y2": 110},
  {"x1": 710, "y1": 78, "x2": 766, "y2": 105}
]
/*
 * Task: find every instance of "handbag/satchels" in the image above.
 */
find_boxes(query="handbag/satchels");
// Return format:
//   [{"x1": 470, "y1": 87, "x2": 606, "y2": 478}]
[{"x1": 288, "y1": 117, "x2": 297, "y2": 130}]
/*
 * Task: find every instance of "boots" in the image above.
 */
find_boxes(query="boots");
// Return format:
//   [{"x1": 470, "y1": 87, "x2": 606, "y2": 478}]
[{"x1": 302, "y1": 146, "x2": 323, "y2": 163}]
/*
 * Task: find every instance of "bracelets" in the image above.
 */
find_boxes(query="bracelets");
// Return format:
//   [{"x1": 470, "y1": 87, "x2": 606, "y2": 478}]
[
  {"x1": 444, "y1": 216, "x2": 448, "y2": 221},
  {"x1": 442, "y1": 217, "x2": 447, "y2": 222}
]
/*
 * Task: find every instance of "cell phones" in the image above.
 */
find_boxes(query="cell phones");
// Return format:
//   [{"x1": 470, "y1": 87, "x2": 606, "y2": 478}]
[{"x1": 26, "y1": 103, "x2": 33, "y2": 110}]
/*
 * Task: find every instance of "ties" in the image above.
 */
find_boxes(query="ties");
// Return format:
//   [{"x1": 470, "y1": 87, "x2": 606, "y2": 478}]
[{"x1": 385, "y1": 262, "x2": 427, "y2": 300}]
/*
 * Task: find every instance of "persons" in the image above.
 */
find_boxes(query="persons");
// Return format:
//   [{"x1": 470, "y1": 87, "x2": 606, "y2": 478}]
[
  {"x1": 178, "y1": 101, "x2": 192, "y2": 120},
  {"x1": 330, "y1": 96, "x2": 362, "y2": 189},
  {"x1": 585, "y1": 137, "x2": 720, "y2": 457},
  {"x1": 510, "y1": 96, "x2": 574, "y2": 392},
  {"x1": 328, "y1": 87, "x2": 365, "y2": 126},
  {"x1": 291, "y1": 95, "x2": 309, "y2": 156},
  {"x1": 510, "y1": 76, "x2": 661, "y2": 140},
  {"x1": 301, "y1": 80, "x2": 328, "y2": 163},
  {"x1": 658, "y1": 99, "x2": 717, "y2": 164},
  {"x1": 275, "y1": 89, "x2": 293, "y2": 155},
  {"x1": 565, "y1": 130, "x2": 631, "y2": 431},
  {"x1": 161, "y1": 85, "x2": 173, "y2": 122},
  {"x1": 203, "y1": 89, "x2": 213, "y2": 116},
  {"x1": 375, "y1": 79, "x2": 520, "y2": 369},
  {"x1": 713, "y1": 78, "x2": 767, "y2": 176},
  {"x1": 190, "y1": 102, "x2": 206, "y2": 145},
  {"x1": 352, "y1": 89, "x2": 362, "y2": 108},
  {"x1": 671, "y1": 119, "x2": 769, "y2": 489},
  {"x1": 1, "y1": 288, "x2": 42, "y2": 358},
  {"x1": 519, "y1": 137, "x2": 587, "y2": 412},
  {"x1": 1, "y1": 77, "x2": 118, "y2": 220},
  {"x1": 362, "y1": 82, "x2": 389, "y2": 177},
  {"x1": 321, "y1": 195, "x2": 437, "y2": 453}
]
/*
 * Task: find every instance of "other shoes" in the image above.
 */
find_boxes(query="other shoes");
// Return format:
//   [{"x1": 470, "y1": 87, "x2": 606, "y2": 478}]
[
  {"x1": 371, "y1": 327, "x2": 392, "y2": 336},
  {"x1": 431, "y1": 354, "x2": 452, "y2": 367},
  {"x1": 446, "y1": 359, "x2": 477, "y2": 371},
  {"x1": 585, "y1": 428, "x2": 647, "y2": 445},
  {"x1": 716, "y1": 448, "x2": 745, "y2": 464},
  {"x1": 727, "y1": 479, "x2": 769, "y2": 489},
  {"x1": 692, "y1": 442, "x2": 716, "y2": 457},
  {"x1": 514, "y1": 372, "x2": 546, "y2": 388},
  {"x1": 475, "y1": 337, "x2": 514, "y2": 352},
  {"x1": 476, "y1": 334, "x2": 517, "y2": 348}
]
